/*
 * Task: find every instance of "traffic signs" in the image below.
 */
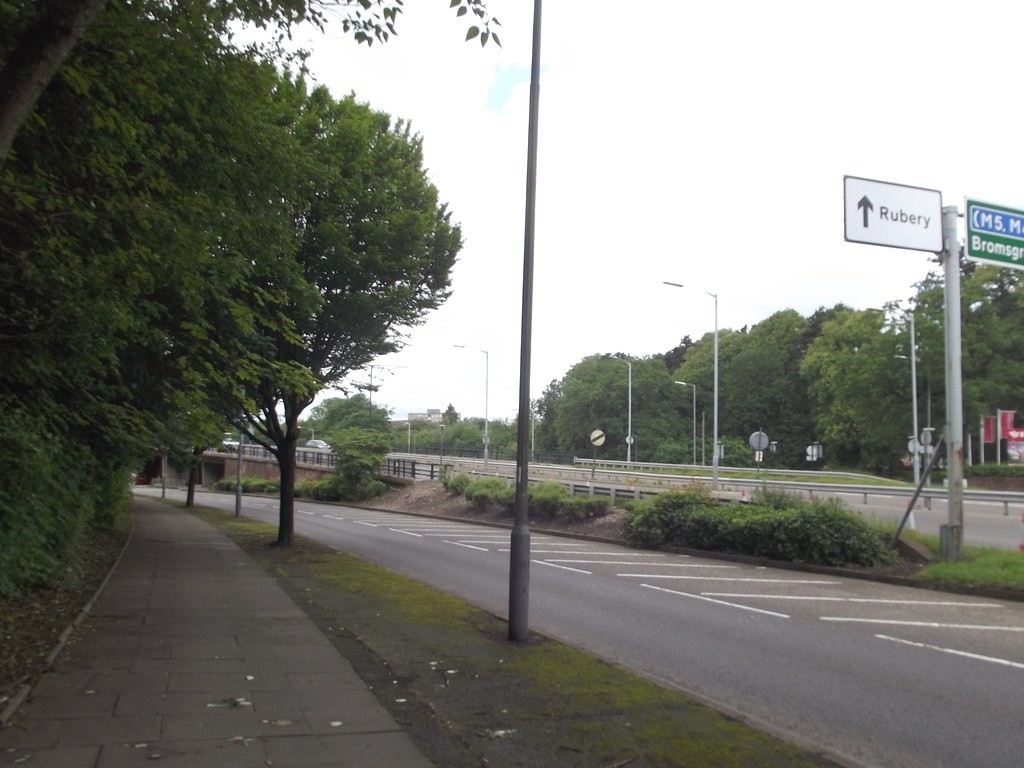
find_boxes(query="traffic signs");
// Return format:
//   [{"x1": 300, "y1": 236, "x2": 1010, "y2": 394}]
[{"x1": 843, "y1": 174, "x2": 944, "y2": 253}]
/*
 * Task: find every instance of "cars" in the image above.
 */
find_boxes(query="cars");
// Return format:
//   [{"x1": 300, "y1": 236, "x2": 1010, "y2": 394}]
[{"x1": 304, "y1": 439, "x2": 331, "y2": 449}]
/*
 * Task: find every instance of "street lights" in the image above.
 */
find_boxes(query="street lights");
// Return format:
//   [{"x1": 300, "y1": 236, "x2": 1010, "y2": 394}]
[
  {"x1": 297, "y1": 426, "x2": 314, "y2": 440},
  {"x1": 674, "y1": 380, "x2": 696, "y2": 466},
  {"x1": 454, "y1": 343, "x2": 489, "y2": 474},
  {"x1": 663, "y1": 280, "x2": 719, "y2": 500},
  {"x1": 610, "y1": 356, "x2": 631, "y2": 462},
  {"x1": 511, "y1": 407, "x2": 534, "y2": 464}
]
